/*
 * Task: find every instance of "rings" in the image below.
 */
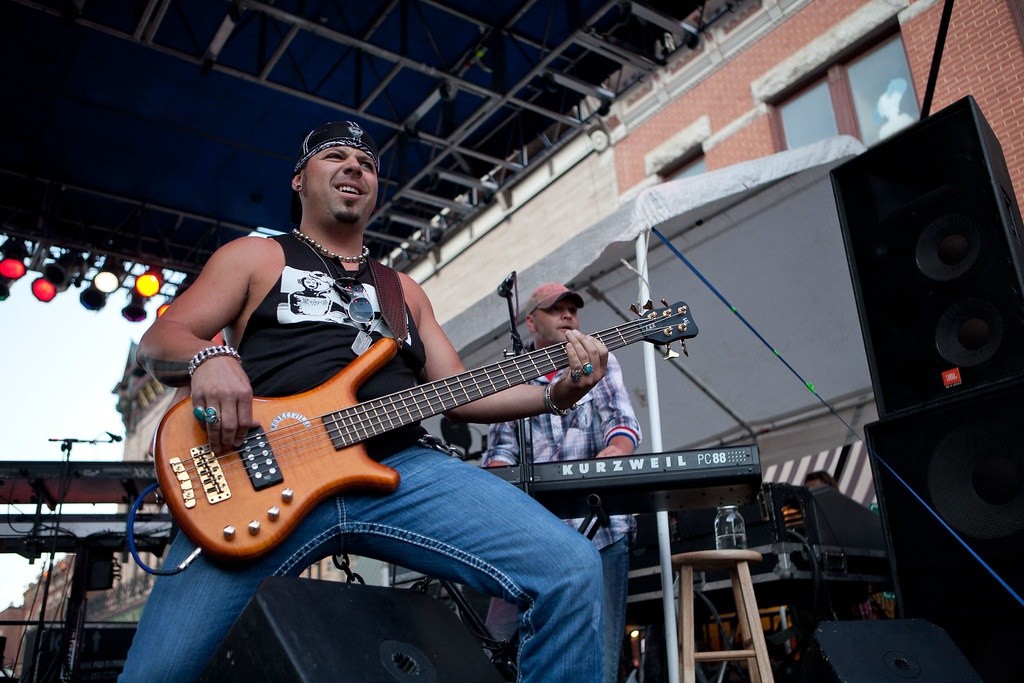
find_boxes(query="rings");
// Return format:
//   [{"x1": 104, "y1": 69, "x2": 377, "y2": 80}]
[
  {"x1": 193, "y1": 406, "x2": 205, "y2": 420},
  {"x1": 569, "y1": 369, "x2": 583, "y2": 379},
  {"x1": 205, "y1": 407, "x2": 222, "y2": 425},
  {"x1": 582, "y1": 362, "x2": 593, "y2": 375}
]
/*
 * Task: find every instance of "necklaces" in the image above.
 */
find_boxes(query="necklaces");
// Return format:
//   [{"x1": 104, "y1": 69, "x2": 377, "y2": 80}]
[{"x1": 292, "y1": 228, "x2": 370, "y2": 263}]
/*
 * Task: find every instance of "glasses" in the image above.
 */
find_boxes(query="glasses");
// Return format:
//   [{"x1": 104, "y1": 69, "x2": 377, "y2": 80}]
[{"x1": 335, "y1": 277, "x2": 375, "y2": 333}]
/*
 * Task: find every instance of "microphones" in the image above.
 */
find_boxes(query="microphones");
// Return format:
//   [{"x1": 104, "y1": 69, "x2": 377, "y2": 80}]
[
  {"x1": 498, "y1": 272, "x2": 516, "y2": 297},
  {"x1": 106, "y1": 431, "x2": 123, "y2": 442}
]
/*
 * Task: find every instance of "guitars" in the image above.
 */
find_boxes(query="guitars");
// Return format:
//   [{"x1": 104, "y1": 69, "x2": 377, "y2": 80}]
[{"x1": 155, "y1": 296, "x2": 700, "y2": 561}]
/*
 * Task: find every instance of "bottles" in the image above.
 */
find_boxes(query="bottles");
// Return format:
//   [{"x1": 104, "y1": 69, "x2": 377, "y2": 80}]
[{"x1": 714, "y1": 505, "x2": 747, "y2": 550}]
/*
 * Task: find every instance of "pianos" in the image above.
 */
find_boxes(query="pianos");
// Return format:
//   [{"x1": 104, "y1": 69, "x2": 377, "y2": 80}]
[{"x1": 476, "y1": 444, "x2": 763, "y2": 683}]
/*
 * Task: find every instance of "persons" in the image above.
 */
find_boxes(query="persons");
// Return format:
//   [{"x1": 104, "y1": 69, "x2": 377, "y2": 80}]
[
  {"x1": 804, "y1": 471, "x2": 838, "y2": 491},
  {"x1": 114, "y1": 121, "x2": 605, "y2": 683},
  {"x1": 480, "y1": 283, "x2": 642, "y2": 683}
]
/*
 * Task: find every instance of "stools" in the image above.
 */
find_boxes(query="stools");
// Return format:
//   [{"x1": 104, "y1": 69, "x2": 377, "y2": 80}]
[{"x1": 672, "y1": 550, "x2": 775, "y2": 682}]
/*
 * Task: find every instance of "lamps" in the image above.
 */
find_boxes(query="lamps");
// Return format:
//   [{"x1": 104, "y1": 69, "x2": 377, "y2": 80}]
[{"x1": 0, "y1": 234, "x2": 190, "y2": 323}]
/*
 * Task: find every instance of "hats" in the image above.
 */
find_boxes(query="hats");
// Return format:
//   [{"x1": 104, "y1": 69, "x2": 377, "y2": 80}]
[
  {"x1": 293, "y1": 119, "x2": 381, "y2": 175},
  {"x1": 526, "y1": 282, "x2": 584, "y2": 315}
]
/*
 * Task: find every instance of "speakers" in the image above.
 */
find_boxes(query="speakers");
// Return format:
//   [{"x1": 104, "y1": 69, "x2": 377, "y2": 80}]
[
  {"x1": 792, "y1": 96, "x2": 1024, "y2": 683},
  {"x1": 197, "y1": 573, "x2": 505, "y2": 683}
]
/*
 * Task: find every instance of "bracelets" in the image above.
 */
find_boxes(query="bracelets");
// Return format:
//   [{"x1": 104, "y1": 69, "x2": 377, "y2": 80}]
[
  {"x1": 188, "y1": 345, "x2": 243, "y2": 376},
  {"x1": 543, "y1": 383, "x2": 577, "y2": 417}
]
